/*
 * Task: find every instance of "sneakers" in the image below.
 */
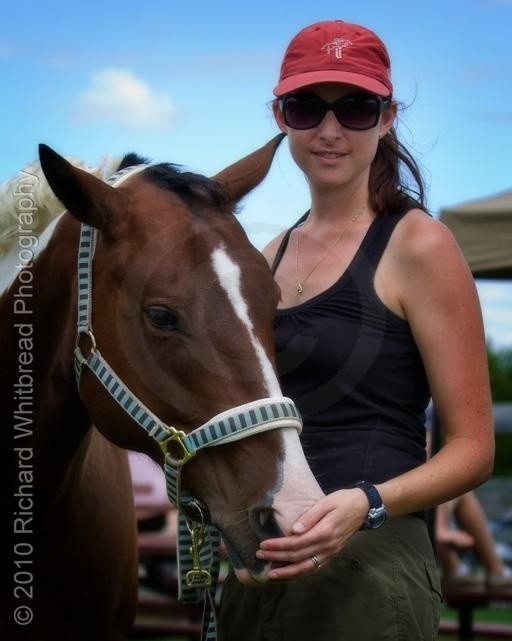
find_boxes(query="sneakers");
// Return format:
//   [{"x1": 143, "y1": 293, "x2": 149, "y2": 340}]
[
  {"x1": 442, "y1": 565, "x2": 469, "y2": 596},
  {"x1": 488, "y1": 568, "x2": 512, "y2": 588}
]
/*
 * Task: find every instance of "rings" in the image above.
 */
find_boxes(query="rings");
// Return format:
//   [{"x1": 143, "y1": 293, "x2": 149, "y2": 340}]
[{"x1": 311, "y1": 556, "x2": 320, "y2": 568}]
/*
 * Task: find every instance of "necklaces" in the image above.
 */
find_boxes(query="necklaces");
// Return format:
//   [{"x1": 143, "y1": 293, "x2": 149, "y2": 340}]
[{"x1": 295, "y1": 199, "x2": 370, "y2": 296}]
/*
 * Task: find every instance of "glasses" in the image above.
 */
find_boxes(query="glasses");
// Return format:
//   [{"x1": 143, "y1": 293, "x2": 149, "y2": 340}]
[{"x1": 282, "y1": 92, "x2": 390, "y2": 131}]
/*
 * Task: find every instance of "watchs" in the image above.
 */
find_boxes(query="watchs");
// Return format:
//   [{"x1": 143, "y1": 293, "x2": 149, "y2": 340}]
[{"x1": 354, "y1": 481, "x2": 387, "y2": 533}]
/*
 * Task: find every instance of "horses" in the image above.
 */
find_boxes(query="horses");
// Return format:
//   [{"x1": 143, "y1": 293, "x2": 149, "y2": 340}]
[{"x1": 0, "y1": 128, "x2": 334, "y2": 640}]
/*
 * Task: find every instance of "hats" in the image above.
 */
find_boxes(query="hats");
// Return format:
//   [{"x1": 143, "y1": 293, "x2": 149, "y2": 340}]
[{"x1": 273, "y1": 18, "x2": 394, "y2": 98}]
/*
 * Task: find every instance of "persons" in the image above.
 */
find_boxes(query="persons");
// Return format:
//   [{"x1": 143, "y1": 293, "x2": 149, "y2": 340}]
[
  {"x1": 207, "y1": 16, "x2": 497, "y2": 641},
  {"x1": 435, "y1": 490, "x2": 510, "y2": 590},
  {"x1": 127, "y1": 448, "x2": 181, "y2": 606}
]
